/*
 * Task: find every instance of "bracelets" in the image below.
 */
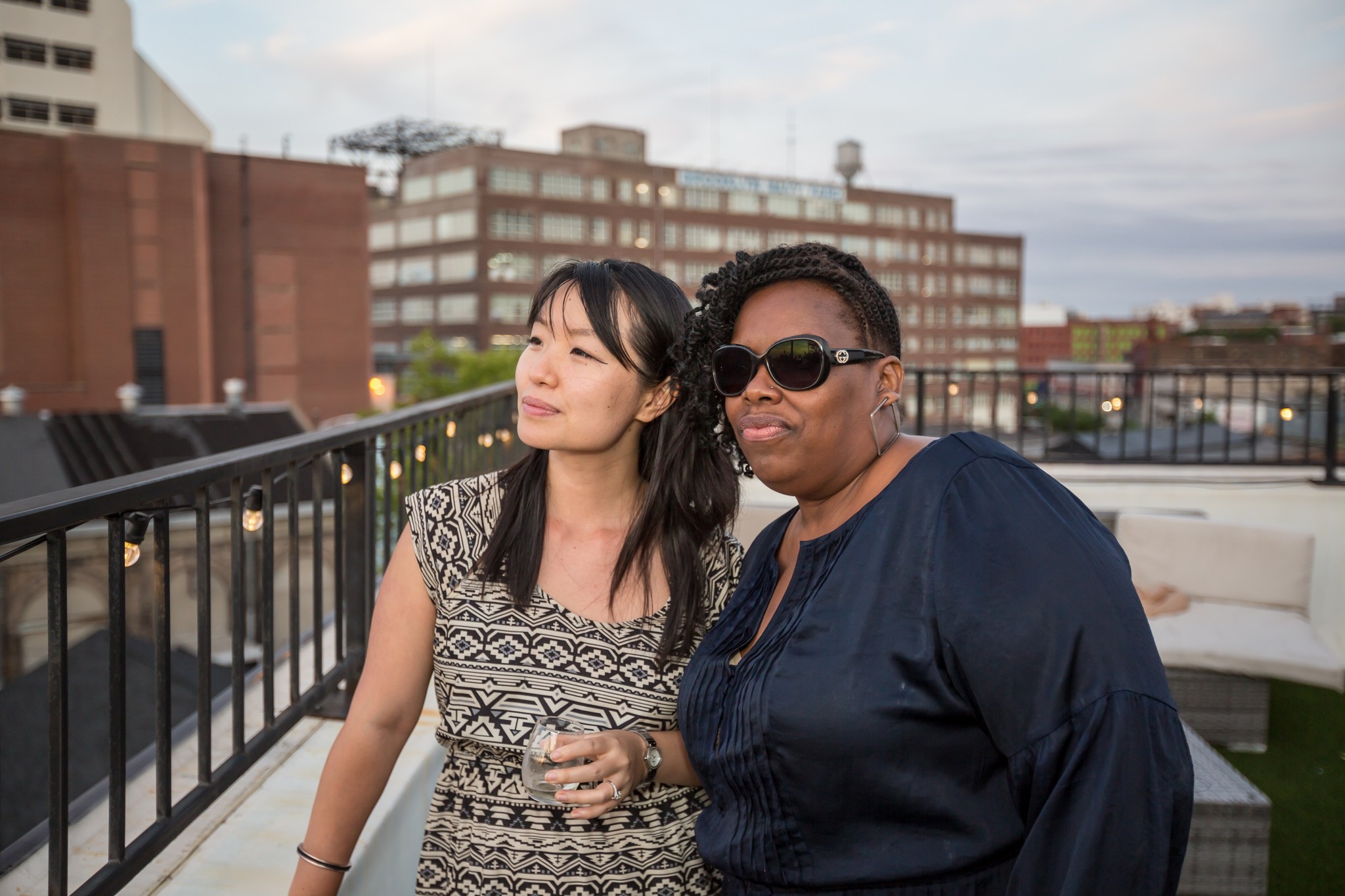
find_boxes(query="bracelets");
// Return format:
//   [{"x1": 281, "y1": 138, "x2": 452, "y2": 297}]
[{"x1": 297, "y1": 843, "x2": 352, "y2": 872}]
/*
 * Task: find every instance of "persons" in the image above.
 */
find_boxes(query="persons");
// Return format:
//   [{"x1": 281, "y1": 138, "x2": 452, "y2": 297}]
[
  {"x1": 289, "y1": 259, "x2": 742, "y2": 896},
  {"x1": 659, "y1": 243, "x2": 1194, "y2": 896}
]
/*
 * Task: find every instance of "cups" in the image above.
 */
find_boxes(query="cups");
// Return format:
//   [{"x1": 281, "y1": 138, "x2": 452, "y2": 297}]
[{"x1": 521, "y1": 715, "x2": 585, "y2": 805}]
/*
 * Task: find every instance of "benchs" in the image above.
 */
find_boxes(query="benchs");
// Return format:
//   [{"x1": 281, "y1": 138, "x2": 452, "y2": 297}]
[{"x1": 1177, "y1": 712, "x2": 1274, "y2": 895}]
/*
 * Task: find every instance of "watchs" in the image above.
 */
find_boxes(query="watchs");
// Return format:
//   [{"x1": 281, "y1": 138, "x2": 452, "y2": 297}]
[{"x1": 629, "y1": 730, "x2": 663, "y2": 789}]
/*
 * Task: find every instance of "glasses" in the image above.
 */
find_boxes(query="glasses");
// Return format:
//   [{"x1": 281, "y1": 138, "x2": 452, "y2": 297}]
[{"x1": 712, "y1": 334, "x2": 887, "y2": 397}]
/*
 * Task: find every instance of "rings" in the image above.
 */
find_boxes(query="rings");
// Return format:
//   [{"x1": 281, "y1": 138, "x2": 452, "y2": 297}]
[{"x1": 605, "y1": 780, "x2": 621, "y2": 799}]
